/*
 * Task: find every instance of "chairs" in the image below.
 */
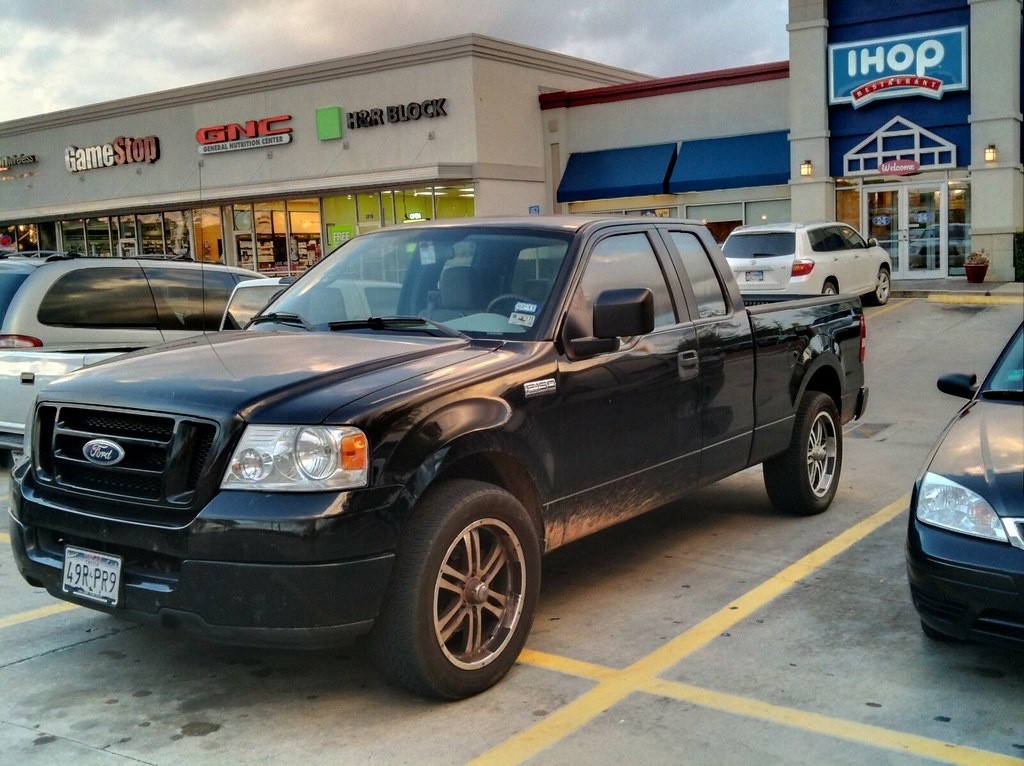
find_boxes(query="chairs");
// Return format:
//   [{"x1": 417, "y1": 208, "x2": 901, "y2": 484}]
[{"x1": 414, "y1": 266, "x2": 480, "y2": 323}]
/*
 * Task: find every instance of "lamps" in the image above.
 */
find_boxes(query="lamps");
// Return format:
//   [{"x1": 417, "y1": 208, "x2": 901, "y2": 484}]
[
  {"x1": 985, "y1": 145, "x2": 997, "y2": 161},
  {"x1": 800, "y1": 160, "x2": 813, "y2": 176}
]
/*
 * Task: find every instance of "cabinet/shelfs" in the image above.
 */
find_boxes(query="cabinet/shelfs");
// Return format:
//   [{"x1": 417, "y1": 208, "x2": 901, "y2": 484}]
[
  {"x1": 235, "y1": 233, "x2": 324, "y2": 277},
  {"x1": 64, "y1": 238, "x2": 180, "y2": 260}
]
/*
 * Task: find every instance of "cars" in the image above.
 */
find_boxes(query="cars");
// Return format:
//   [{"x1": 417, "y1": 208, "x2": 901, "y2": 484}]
[
  {"x1": 905, "y1": 316, "x2": 1023, "y2": 652},
  {"x1": 909, "y1": 222, "x2": 968, "y2": 266}
]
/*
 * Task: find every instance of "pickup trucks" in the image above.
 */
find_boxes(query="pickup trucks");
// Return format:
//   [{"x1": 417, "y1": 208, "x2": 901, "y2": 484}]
[
  {"x1": 0, "y1": 274, "x2": 400, "y2": 450},
  {"x1": 4, "y1": 211, "x2": 871, "y2": 700}
]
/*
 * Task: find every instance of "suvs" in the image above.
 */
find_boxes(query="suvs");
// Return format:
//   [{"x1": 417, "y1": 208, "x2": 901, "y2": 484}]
[
  {"x1": 1, "y1": 256, "x2": 269, "y2": 348},
  {"x1": 722, "y1": 219, "x2": 894, "y2": 308}
]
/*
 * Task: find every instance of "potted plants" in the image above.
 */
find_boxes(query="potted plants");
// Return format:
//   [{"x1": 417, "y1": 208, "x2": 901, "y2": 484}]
[{"x1": 963, "y1": 249, "x2": 991, "y2": 283}]
